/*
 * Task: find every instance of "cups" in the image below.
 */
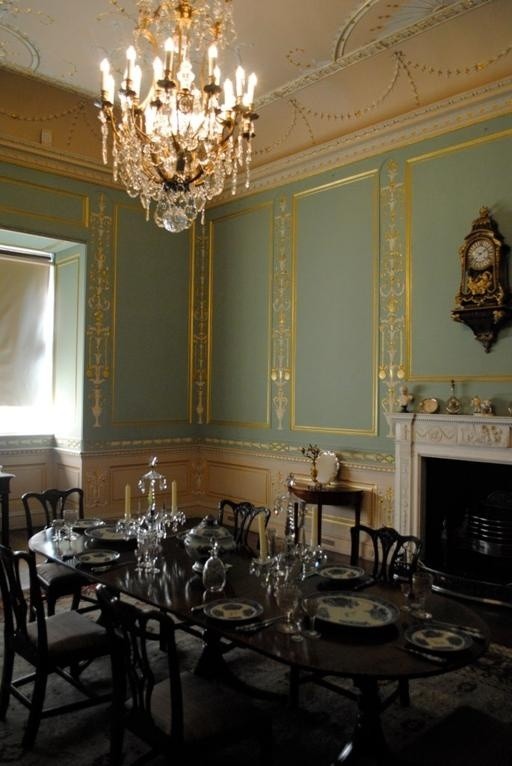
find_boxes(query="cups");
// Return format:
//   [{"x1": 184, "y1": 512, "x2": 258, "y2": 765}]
[{"x1": 51, "y1": 518, "x2": 65, "y2": 542}]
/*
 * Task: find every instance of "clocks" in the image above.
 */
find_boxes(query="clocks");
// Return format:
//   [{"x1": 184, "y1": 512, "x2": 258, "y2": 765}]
[{"x1": 450, "y1": 203, "x2": 512, "y2": 353}]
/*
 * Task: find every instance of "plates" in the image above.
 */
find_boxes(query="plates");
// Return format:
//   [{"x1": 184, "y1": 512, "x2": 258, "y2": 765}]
[
  {"x1": 302, "y1": 591, "x2": 401, "y2": 629},
  {"x1": 84, "y1": 523, "x2": 125, "y2": 541},
  {"x1": 204, "y1": 598, "x2": 263, "y2": 620},
  {"x1": 314, "y1": 563, "x2": 365, "y2": 582},
  {"x1": 70, "y1": 517, "x2": 105, "y2": 529},
  {"x1": 418, "y1": 397, "x2": 440, "y2": 412},
  {"x1": 74, "y1": 549, "x2": 121, "y2": 564},
  {"x1": 175, "y1": 528, "x2": 191, "y2": 541},
  {"x1": 403, "y1": 623, "x2": 474, "y2": 655},
  {"x1": 314, "y1": 450, "x2": 340, "y2": 485}
]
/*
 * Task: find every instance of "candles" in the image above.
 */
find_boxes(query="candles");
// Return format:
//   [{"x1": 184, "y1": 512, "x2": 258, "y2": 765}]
[
  {"x1": 309, "y1": 506, "x2": 316, "y2": 547},
  {"x1": 171, "y1": 480, "x2": 178, "y2": 513},
  {"x1": 258, "y1": 512, "x2": 268, "y2": 562},
  {"x1": 124, "y1": 483, "x2": 131, "y2": 517}
]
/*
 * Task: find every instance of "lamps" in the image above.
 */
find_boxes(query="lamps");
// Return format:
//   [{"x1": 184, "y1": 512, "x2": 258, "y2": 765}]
[{"x1": 92, "y1": 1, "x2": 258, "y2": 234}]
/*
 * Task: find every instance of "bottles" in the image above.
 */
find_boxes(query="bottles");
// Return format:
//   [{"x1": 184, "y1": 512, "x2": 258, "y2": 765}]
[
  {"x1": 201, "y1": 535, "x2": 227, "y2": 593},
  {"x1": 444, "y1": 380, "x2": 462, "y2": 414},
  {"x1": 202, "y1": 590, "x2": 223, "y2": 604}
]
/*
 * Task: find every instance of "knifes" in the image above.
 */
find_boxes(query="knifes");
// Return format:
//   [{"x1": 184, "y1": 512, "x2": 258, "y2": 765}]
[
  {"x1": 91, "y1": 560, "x2": 137, "y2": 573},
  {"x1": 235, "y1": 613, "x2": 289, "y2": 633},
  {"x1": 422, "y1": 618, "x2": 485, "y2": 639},
  {"x1": 390, "y1": 642, "x2": 448, "y2": 665}
]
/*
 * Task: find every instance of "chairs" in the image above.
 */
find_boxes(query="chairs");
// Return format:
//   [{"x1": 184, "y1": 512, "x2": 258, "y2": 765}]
[
  {"x1": 20, "y1": 487, "x2": 100, "y2": 622},
  {"x1": 159, "y1": 500, "x2": 271, "y2": 652},
  {"x1": 95, "y1": 584, "x2": 271, "y2": 765},
  {"x1": 0, "y1": 546, "x2": 127, "y2": 763},
  {"x1": 286, "y1": 524, "x2": 421, "y2": 745}
]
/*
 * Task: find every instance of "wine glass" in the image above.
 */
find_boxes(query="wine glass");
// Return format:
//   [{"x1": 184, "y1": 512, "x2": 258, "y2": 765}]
[
  {"x1": 63, "y1": 509, "x2": 79, "y2": 543},
  {"x1": 399, "y1": 572, "x2": 435, "y2": 621},
  {"x1": 276, "y1": 580, "x2": 321, "y2": 643}
]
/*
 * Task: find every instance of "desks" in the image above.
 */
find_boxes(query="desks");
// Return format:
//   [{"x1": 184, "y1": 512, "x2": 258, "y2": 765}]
[{"x1": 290, "y1": 480, "x2": 363, "y2": 564}]
